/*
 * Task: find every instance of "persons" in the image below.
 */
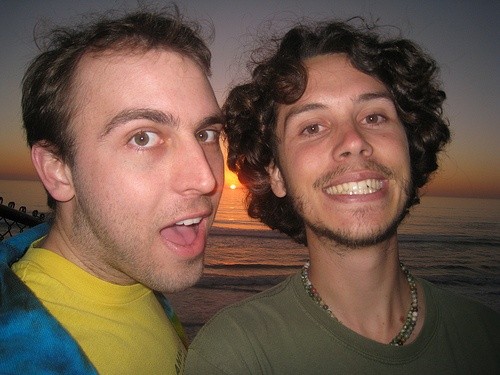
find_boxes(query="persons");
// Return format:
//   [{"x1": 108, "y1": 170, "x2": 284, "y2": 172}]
[
  {"x1": 182, "y1": 15, "x2": 500, "y2": 375},
  {"x1": 0, "y1": 0, "x2": 225, "y2": 375}
]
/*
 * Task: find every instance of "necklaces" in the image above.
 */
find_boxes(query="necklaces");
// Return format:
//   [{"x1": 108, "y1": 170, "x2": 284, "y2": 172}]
[{"x1": 301, "y1": 259, "x2": 419, "y2": 346}]
